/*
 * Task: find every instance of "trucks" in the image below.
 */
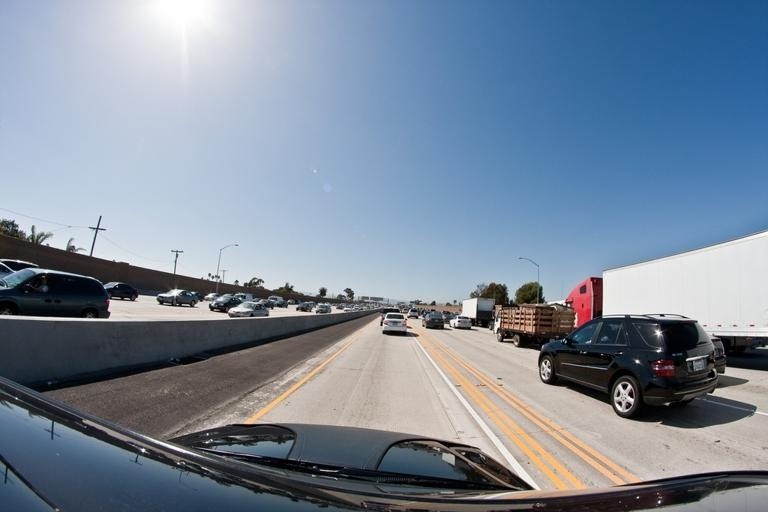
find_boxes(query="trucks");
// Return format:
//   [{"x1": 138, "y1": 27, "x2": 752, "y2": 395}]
[{"x1": 563, "y1": 229, "x2": 768, "y2": 353}]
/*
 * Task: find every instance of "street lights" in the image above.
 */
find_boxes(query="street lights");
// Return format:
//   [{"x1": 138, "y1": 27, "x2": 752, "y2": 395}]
[
  {"x1": 216, "y1": 241, "x2": 240, "y2": 280},
  {"x1": 517, "y1": 256, "x2": 541, "y2": 304}
]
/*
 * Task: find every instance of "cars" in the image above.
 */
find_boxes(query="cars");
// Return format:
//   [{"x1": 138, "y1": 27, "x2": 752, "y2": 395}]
[{"x1": 0, "y1": 408, "x2": 767, "y2": 512}]
